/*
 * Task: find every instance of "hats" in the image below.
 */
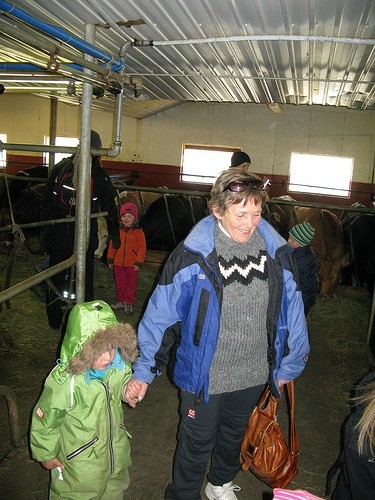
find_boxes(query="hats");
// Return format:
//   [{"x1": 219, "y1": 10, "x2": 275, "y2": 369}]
[
  {"x1": 230, "y1": 151, "x2": 251, "y2": 167},
  {"x1": 120, "y1": 202, "x2": 138, "y2": 218},
  {"x1": 288, "y1": 221, "x2": 315, "y2": 245}
]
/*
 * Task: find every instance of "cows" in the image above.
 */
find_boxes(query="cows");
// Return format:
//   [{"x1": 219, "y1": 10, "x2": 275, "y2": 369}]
[
  {"x1": 260, "y1": 196, "x2": 294, "y2": 239},
  {"x1": 345, "y1": 202, "x2": 374, "y2": 289},
  {"x1": 89, "y1": 189, "x2": 213, "y2": 259},
  {"x1": 294, "y1": 203, "x2": 351, "y2": 302}
]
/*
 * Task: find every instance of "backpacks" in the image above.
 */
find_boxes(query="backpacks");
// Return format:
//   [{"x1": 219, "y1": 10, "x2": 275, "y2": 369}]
[{"x1": 53, "y1": 158, "x2": 94, "y2": 210}]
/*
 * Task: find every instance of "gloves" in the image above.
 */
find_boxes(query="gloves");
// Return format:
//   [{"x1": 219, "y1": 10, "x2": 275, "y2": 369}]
[{"x1": 106, "y1": 221, "x2": 122, "y2": 249}]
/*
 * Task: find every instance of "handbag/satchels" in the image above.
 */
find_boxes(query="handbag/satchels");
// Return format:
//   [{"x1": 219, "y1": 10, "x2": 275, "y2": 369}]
[{"x1": 241, "y1": 377, "x2": 301, "y2": 489}]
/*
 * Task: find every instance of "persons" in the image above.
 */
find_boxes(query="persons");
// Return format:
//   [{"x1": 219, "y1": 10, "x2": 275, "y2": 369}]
[
  {"x1": 325, "y1": 370, "x2": 375, "y2": 500},
  {"x1": 106, "y1": 202, "x2": 146, "y2": 315},
  {"x1": 126, "y1": 168, "x2": 310, "y2": 500},
  {"x1": 40, "y1": 130, "x2": 121, "y2": 329},
  {"x1": 29, "y1": 300, "x2": 142, "y2": 500},
  {"x1": 229, "y1": 151, "x2": 251, "y2": 171},
  {"x1": 287, "y1": 220, "x2": 320, "y2": 318}
]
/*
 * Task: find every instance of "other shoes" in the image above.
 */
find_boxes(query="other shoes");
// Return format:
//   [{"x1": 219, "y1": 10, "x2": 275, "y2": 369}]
[
  {"x1": 203, "y1": 481, "x2": 239, "y2": 500},
  {"x1": 111, "y1": 301, "x2": 124, "y2": 310},
  {"x1": 124, "y1": 303, "x2": 133, "y2": 314},
  {"x1": 30, "y1": 282, "x2": 46, "y2": 301}
]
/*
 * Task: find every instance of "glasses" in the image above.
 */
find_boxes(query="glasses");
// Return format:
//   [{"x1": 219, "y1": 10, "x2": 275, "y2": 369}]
[{"x1": 222, "y1": 178, "x2": 265, "y2": 193}]
